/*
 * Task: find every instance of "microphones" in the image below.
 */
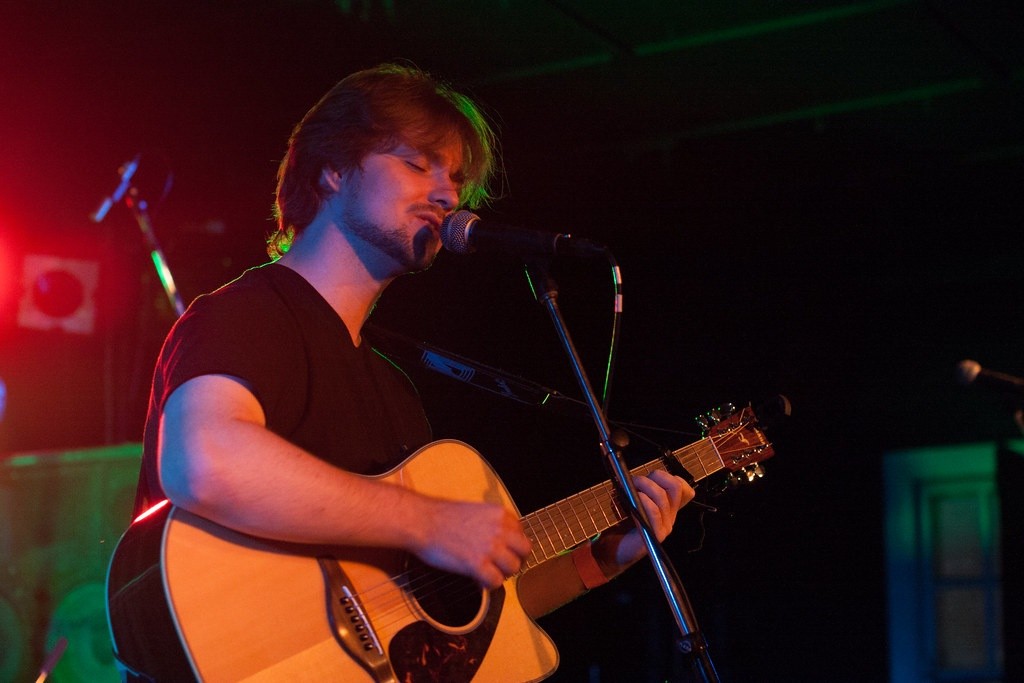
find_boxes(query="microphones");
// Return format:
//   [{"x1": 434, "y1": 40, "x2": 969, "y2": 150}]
[
  {"x1": 441, "y1": 210, "x2": 607, "y2": 254},
  {"x1": 36, "y1": 636, "x2": 68, "y2": 683},
  {"x1": 956, "y1": 359, "x2": 1024, "y2": 393},
  {"x1": 91, "y1": 155, "x2": 143, "y2": 220}
]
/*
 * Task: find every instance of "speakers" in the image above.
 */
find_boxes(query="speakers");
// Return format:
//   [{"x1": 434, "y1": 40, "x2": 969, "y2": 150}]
[{"x1": 0, "y1": 442, "x2": 144, "y2": 683}]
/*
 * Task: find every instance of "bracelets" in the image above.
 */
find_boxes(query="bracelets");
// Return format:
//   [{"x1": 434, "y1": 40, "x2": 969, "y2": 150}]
[{"x1": 569, "y1": 540, "x2": 610, "y2": 591}]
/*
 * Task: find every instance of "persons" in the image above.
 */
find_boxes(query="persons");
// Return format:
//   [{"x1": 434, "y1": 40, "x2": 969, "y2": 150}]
[{"x1": 126, "y1": 64, "x2": 696, "y2": 683}]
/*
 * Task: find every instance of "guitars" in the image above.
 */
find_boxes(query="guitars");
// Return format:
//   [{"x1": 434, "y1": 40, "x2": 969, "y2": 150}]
[{"x1": 105, "y1": 394, "x2": 793, "y2": 682}]
